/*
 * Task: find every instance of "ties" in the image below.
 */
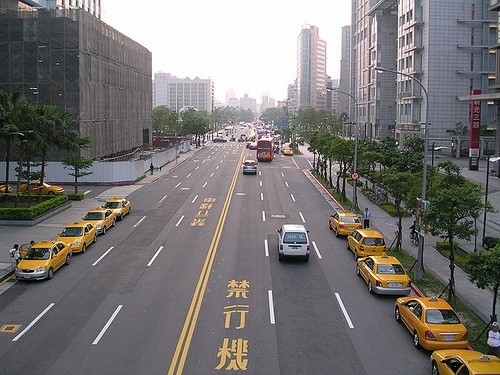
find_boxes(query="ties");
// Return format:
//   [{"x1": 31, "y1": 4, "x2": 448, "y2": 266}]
[{"x1": 366, "y1": 211, "x2": 367, "y2": 218}]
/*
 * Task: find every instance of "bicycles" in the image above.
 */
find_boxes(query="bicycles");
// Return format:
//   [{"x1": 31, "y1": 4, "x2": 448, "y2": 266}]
[{"x1": 409, "y1": 228, "x2": 419, "y2": 246}]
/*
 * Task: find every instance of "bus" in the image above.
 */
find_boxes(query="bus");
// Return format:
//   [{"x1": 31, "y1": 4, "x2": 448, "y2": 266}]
[{"x1": 256, "y1": 138, "x2": 275, "y2": 161}]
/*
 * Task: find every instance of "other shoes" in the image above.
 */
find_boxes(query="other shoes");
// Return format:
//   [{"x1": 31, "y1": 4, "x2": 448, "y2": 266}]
[{"x1": 411, "y1": 237, "x2": 413, "y2": 239}]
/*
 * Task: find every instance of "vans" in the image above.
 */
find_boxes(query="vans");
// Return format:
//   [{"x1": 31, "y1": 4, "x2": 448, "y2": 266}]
[{"x1": 276, "y1": 224, "x2": 310, "y2": 262}]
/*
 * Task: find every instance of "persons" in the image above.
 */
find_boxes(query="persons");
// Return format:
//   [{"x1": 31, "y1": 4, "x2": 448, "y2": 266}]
[
  {"x1": 487, "y1": 322, "x2": 500, "y2": 354},
  {"x1": 150, "y1": 163, "x2": 154, "y2": 175},
  {"x1": 363, "y1": 207, "x2": 370, "y2": 229},
  {"x1": 27, "y1": 240, "x2": 35, "y2": 249},
  {"x1": 9, "y1": 244, "x2": 20, "y2": 265},
  {"x1": 409, "y1": 220, "x2": 420, "y2": 245}
]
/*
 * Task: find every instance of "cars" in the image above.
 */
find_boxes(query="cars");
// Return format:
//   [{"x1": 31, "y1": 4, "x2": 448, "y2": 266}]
[
  {"x1": 346, "y1": 227, "x2": 388, "y2": 261},
  {"x1": 430, "y1": 349, "x2": 500, "y2": 375},
  {"x1": 242, "y1": 160, "x2": 258, "y2": 175},
  {"x1": 14, "y1": 240, "x2": 72, "y2": 281},
  {"x1": 213, "y1": 122, "x2": 293, "y2": 155},
  {"x1": 101, "y1": 197, "x2": 132, "y2": 221},
  {"x1": 394, "y1": 297, "x2": 469, "y2": 351},
  {"x1": 81, "y1": 208, "x2": 116, "y2": 235},
  {"x1": 0, "y1": 184, "x2": 13, "y2": 193},
  {"x1": 355, "y1": 253, "x2": 412, "y2": 296},
  {"x1": 18, "y1": 180, "x2": 65, "y2": 195},
  {"x1": 57, "y1": 221, "x2": 98, "y2": 254},
  {"x1": 329, "y1": 212, "x2": 363, "y2": 237}
]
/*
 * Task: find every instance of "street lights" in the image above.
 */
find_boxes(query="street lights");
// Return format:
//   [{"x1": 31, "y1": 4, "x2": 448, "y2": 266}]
[
  {"x1": 482, "y1": 139, "x2": 496, "y2": 247},
  {"x1": 373, "y1": 67, "x2": 429, "y2": 201},
  {"x1": 325, "y1": 87, "x2": 359, "y2": 211}
]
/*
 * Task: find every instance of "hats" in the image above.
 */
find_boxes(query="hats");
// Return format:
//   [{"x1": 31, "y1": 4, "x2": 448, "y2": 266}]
[{"x1": 493, "y1": 322, "x2": 499, "y2": 327}]
